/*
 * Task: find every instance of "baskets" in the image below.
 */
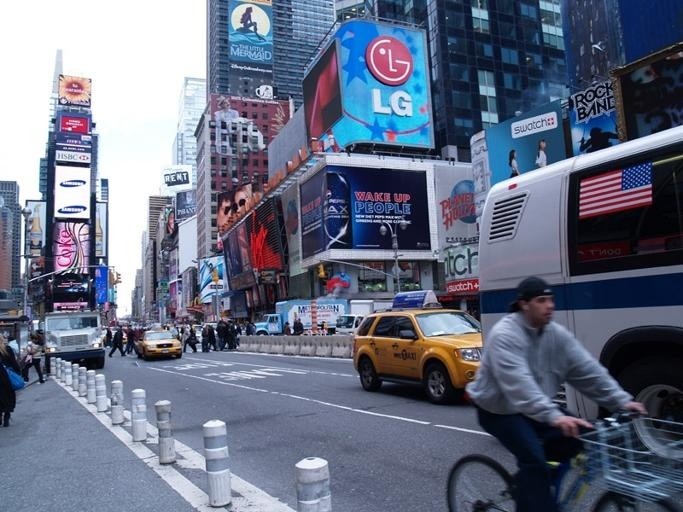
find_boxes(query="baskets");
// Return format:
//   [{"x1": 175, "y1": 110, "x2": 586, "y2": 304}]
[{"x1": 579, "y1": 417, "x2": 683, "y2": 503}]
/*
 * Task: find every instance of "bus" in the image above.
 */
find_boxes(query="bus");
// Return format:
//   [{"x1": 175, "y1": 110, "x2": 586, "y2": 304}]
[{"x1": 477, "y1": 123, "x2": 683, "y2": 464}]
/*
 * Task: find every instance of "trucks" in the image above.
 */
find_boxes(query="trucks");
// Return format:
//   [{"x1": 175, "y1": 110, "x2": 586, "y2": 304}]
[
  {"x1": 43, "y1": 307, "x2": 106, "y2": 373},
  {"x1": 251, "y1": 297, "x2": 398, "y2": 336}
]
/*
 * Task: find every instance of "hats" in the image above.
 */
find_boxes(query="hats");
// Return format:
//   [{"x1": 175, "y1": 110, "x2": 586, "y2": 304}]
[{"x1": 508, "y1": 278, "x2": 553, "y2": 311}]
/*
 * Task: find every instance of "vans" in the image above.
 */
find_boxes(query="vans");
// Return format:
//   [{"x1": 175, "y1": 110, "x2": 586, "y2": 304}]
[{"x1": 353, "y1": 289, "x2": 487, "y2": 402}]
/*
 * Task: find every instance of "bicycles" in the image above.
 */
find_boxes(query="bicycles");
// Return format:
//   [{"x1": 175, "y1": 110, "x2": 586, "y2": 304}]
[{"x1": 445, "y1": 409, "x2": 682, "y2": 511}]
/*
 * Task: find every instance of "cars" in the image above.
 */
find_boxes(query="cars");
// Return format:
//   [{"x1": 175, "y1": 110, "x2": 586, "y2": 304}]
[
  {"x1": 175, "y1": 325, "x2": 207, "y2": 342},
  {"x1": 134, "y1": 323, "x2": 182, "y2": 360},
  {"x1": 107, "y1": 327, "x2": 128, "y2": 344}
]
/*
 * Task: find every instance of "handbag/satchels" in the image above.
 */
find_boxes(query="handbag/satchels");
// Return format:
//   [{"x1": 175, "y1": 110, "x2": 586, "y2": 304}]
[{"x1": 6, "y1": 365, "x2": 24, "y2": 390}]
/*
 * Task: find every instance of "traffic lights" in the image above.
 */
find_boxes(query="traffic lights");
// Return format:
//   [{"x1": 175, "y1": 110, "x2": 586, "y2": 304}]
[{"x1": 115, "y1": 272, "x2": 121, "y2": 284}]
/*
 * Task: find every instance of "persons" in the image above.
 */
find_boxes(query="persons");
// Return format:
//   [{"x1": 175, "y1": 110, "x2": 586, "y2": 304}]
[
  {"x1": 534, "y1": 139, "x2": 548, "y2": 166},
  {"x1": 464, "y1": 275, "x2": 649, "y2": 512},
  {"x1": 317, "y1": 319, "x2": 329, "y2": 336},
  {"x1": 166, "y1": 212, "x2": 175, "y2": 233},
  {"x1": 104, "y1": 317, "x2": 256, "y2": 357},
  {"x1": 280, "y1": 316, "x2": 303, "y2": 334},
  {"x1": 508, "y1": 150, "x2": 522, "y2": 177},
  {"x1": 213, "y1": 96, "x2": 268, "y2": 152},
  {"x1": 217, "y1": 191, "x2": 235, "y2": 230},
  {"x1": 0, "y1": 330, "x2": 46, "y2": 427},
  {"x1": 233, "y1": 184, "x2": 251, "y2": 217}
]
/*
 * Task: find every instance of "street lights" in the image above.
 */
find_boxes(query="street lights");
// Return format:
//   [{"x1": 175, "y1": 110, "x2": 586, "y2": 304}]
[
  {"x1": 21, "y1": 205, "x2": 33, "y2": 317},
  {"x1": 377, "y1": 215, "x2": 410, "y2": 294},
  {"x1": 191, "y1": 258, "x2": 220, "y2": 322}
]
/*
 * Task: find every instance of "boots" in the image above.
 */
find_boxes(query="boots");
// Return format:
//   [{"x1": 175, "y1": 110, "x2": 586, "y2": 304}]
[{"x1": 4, "y1": 412, "x2": 10, "y2": 426}]
